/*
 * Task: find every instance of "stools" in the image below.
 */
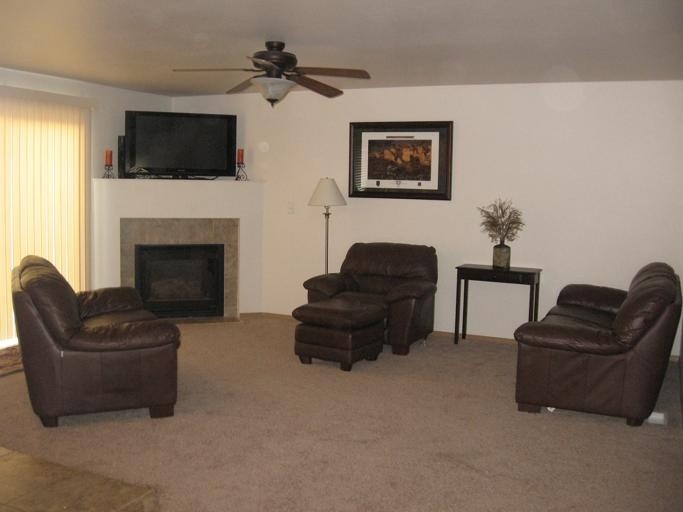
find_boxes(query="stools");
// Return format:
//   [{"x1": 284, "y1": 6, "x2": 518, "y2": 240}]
[{"x1": 293, "y1": 299, "x2": 385, "y2": 371}]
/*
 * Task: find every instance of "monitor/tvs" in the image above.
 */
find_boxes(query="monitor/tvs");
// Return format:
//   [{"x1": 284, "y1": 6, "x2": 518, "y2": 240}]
[{"x1": 125, "y1": 111, "x2": 237, "y2": 180}]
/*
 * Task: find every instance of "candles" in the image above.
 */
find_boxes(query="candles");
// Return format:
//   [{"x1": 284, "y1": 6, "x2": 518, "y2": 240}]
[
  {"x1": 105, "y1": 150, "x2": 112, "y2": 166},
  {"x1": 238, "y1": 149, "x2": 243, "y2": 164}
]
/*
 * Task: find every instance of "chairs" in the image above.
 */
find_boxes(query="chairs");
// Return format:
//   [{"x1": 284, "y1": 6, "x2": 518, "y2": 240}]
[
  {"x1": 304, "y1": 243, "x2": 438, "y2": 355},
  {"x1": 10, "y1": 256, "x2": 181, "y2": 427}
]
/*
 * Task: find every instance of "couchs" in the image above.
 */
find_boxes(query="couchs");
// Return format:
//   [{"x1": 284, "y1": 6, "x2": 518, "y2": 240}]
[{"x1": 513, "y1": 260, "x2": 682, "y2": 427}]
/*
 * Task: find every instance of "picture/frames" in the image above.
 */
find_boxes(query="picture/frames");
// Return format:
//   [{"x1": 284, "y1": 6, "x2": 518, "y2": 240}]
[{"x1": 349, "y1": 121, "x2": 453, "y2": 200}]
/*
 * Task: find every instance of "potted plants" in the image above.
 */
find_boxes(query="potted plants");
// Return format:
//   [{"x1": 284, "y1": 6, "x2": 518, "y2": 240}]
[{"x1": 477, "y1": 197, "x2": 527, "y2": 269}]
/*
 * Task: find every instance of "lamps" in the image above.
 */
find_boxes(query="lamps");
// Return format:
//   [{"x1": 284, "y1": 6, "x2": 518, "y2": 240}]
[
  {"x1": 307, "y1": 177, "x2": 346, "y2": 275},
  {"x1": 250, "y1": 77, "x2": 296, "y2": 107}
]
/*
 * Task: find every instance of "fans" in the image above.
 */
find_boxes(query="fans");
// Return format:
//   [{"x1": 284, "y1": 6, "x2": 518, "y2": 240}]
[{"x1": 172, "y1": 41, "x2": 371, "y2": 98}]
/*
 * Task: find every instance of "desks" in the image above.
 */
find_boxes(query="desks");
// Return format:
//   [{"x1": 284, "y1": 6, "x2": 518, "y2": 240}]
[{"x1": 455, "y1": 264, "x2": 542, "y2": 344}]
